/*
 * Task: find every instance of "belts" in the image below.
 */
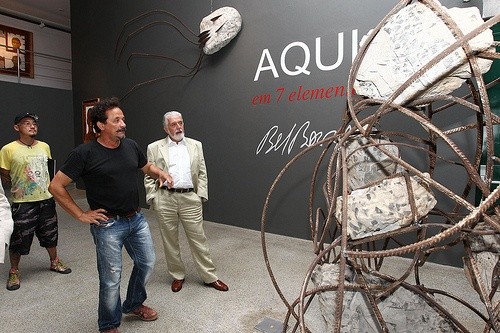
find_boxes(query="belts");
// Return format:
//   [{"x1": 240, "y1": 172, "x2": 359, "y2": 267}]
[
  {"x1": 107, "y1": 207, "x2": 141, "y2": 218},
  {"x1": 158, "y1": 185, "x2": 194, "y2": 192}
]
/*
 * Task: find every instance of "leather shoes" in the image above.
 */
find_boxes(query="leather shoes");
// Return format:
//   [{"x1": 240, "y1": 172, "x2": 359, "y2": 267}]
[
  {"x1": 204, "y1": 280, "x2": 228, "y2": 291},
  {"x1": 172, "y1": 277, "x2": 185, "y2": 292},
  {"x1": 100, "y1": 327, "x2": 119, "y2": 333},
  {"x1": 123, "y1": 304, "x2": 158, "y2": 320}
]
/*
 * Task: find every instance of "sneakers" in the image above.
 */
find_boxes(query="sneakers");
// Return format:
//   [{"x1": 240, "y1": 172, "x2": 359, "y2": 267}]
[
  {"x1": 7, "y1": 270, "x2": 20, "y2": 290},
  {"x1": 50, "y1": 259, "x2": 72, "y2": 274}
]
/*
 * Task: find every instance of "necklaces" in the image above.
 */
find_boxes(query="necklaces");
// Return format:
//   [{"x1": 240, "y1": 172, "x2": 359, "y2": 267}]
[{"x1": 19, "y1": 139, "x2": 34, "y2": 148}]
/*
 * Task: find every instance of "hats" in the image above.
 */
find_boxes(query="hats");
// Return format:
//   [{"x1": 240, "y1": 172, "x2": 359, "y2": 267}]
[{"x1": 14, "y1": 112, "x2": 39, "y2": 124}]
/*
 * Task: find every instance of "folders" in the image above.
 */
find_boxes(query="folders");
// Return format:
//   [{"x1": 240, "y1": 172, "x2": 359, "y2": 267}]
[{"x1": 48, "y1": 157, "x2": 56, "y2": 184}]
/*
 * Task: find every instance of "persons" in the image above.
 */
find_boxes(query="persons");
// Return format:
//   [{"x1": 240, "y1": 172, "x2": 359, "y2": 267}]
[
  {"x1": 144, "y1": 110, "x2": 229, "y2": 293},
  {"x1": 0, "y1": 177, "x2": 14, "y2": 264},
  {"x1": 0, "y1": 112, "x2": 72, "y2": 291},
  {"x1": 48, "y1": 99, "x2": 174, "y2": 333}
]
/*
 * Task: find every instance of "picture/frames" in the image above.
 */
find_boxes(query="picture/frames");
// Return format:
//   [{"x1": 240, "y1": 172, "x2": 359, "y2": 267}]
[{"x1": 0, "y1": 24, "x2": 35, "y2": 80}]
